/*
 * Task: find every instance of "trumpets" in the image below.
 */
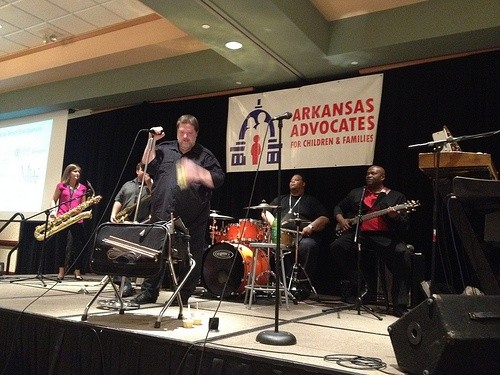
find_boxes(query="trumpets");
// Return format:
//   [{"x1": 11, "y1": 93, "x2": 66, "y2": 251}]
[{"x1": 110, "y1": 193, "x2": 152, "y2": 223}]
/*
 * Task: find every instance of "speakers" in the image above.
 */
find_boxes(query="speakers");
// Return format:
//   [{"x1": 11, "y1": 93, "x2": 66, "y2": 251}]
[{"x1": 387, "y1": 295, "x2": 500, "y2": 375}]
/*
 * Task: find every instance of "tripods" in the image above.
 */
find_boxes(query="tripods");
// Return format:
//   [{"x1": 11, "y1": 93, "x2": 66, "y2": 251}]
[
  {"x1": 240, "y1": 222, "x2": 321, "y2": 305},
  {"x1": 9, "y1": 194, "x2": 87, "y2": 287},
  {"x1": 322, "y1": 190, "x2": 382, "y2": 321}
]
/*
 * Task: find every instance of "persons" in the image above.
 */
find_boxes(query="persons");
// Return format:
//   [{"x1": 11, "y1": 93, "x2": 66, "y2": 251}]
[
  {"x1": 262, "y1": 174, "x2": 331, "y2": 299},
  {"x1": 110, "y1": 162, "x2": 153, "y2": 297},
  {"x1": 131, "y1": 114, "x2": 225, "y2": 304},
  {"x1": 50, "y1": 164, "x2": 100, "y2": 280},
  {"x1": 333, "y1": 166, "x2": 412, "y2": 317}
]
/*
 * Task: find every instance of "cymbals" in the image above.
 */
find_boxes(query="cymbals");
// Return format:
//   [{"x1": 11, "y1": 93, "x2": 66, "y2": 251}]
[
  {"x1": 285, "y1": 217, "x2": 312, "y2": 224},
  {"x1": 243, "y1": 203, "x2": 282, "y2": 209},
  {"x1": 209, "y1": 212, "x2": 235, "y2": 221}
]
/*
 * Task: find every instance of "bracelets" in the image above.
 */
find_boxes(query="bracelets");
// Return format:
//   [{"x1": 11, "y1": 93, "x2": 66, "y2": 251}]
[{"x1": 308, "y1": 224, "x2": 315, "y2": 232}]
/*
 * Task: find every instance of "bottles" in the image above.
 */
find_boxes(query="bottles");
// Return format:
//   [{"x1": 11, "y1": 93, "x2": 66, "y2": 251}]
[{"x1": 271, "y1": 212, "x2": 277, "y2": 244}]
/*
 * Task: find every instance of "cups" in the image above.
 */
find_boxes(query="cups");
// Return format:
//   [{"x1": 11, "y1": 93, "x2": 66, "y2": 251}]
[{"x1": 182, "y1": 302, "x2": 202, "y2": 328}]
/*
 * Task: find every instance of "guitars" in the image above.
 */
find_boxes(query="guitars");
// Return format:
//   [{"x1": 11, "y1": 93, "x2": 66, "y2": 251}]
[{"x1": 333, "y1": 200, "x2": 422, "y2": 240}]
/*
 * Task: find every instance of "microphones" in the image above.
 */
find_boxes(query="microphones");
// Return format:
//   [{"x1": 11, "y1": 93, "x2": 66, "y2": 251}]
[
  {"x1": 86, "y1": 190, "x2": 91, "y2": 196},
  {"x1": 362, "y1": 185, "x2": 367, "y2": 191},
  {"x1": 141, "y1": 129, "x2": 164, "y2": 134},
  {"x1": 272, "y1": 112, "x2": 292, "y2": 121}
]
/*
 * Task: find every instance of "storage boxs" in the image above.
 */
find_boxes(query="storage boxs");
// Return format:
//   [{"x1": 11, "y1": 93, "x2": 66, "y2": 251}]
[{"x1": 386, "y1": 294, "x2": 500, "y2": 375}]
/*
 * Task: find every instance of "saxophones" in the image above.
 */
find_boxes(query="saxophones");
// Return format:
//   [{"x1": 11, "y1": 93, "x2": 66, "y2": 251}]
[{"x1": 33, "y1": 180, "x2": 102, "y2": 242}]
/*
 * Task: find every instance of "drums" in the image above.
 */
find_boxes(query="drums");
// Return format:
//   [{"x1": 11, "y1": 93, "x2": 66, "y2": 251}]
[
  {"x1": 268, "y1": 225, "x2": 293, "y2": 248},
  {"x1": 238, "y1": 218, "x2": 267, "y2": 242},
  {"x1": 215, "y1": 223, "x2": 241, "y2": 242},
  {"x1": 201, "y1": 240, "x2": 270, "y2": 298}
]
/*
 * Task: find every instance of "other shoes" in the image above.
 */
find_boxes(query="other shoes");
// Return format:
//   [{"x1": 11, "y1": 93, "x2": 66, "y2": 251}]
[
  {"x1": 114, "y1": 283, "x2": 136, "y2": 297},
  {"x1": 131, "y1": 292, "x2": 157, "y2": 304},
  {"x1": 57, "y1": 276, "x2": 64, "y2": 280},
  {"x1": 75, "y1": 275, "x2": 83, "y2": 281},
  {"x1": 393, "y1": 305, "x2": 409, "y2": 317},
  {"x1": 354, "y1": 283, "x2": 372, "y2": 302},
  {"x1": 165, "y1": 297, "x2": 188, "y2": 307}
]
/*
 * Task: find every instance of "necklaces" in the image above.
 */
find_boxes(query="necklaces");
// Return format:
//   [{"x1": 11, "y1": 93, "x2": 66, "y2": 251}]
[{"x1": 288, "y1": 194, "x2": 302, "y2": 213}]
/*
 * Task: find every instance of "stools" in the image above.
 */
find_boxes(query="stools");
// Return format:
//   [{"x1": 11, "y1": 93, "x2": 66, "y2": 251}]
[{"x1": 244, "y1": 243, "x2": 290, "y2": 310}]
